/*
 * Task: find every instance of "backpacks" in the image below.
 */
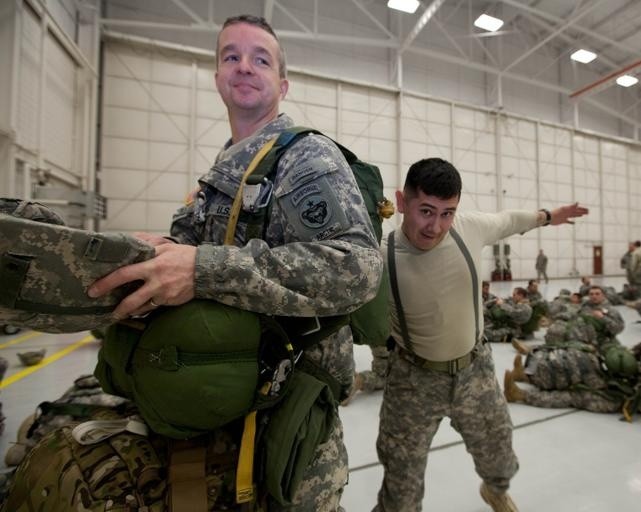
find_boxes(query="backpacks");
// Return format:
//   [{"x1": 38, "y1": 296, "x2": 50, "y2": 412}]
[
  {"x1": 529, "y1": 347, "x2": 608, "y2": 391},
  {"x1": 521, "y1": 303, "x2": 546, "y2": 335},
  {"x1": 1, "y1": 372, "x2": 245, "y2": 512},
  {"x1": 247, "y1": 127, "x2": 396, "y2": 355}
]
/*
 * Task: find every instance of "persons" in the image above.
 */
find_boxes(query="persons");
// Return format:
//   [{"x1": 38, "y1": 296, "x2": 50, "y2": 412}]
[
  {"x1": 341, "y1": 370, "x2": 385, "y2": 406},
  {"x1": 372, "y1": 156, "x2": 589, "y2": 512},
  {"x1": 85, "y1": 12, "x2": 385, "y2": 511},
  {"x1": 481, "y1": 240, "x2": 640, "y2": 415}
]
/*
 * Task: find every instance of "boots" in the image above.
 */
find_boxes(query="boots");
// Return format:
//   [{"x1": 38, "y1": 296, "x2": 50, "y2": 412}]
[
  {"x1": 504, "y1": 336, "x2": 531, "y2": 405},
  {"x1": 479, "y1": 481, "x2": 520, "y2": 512}
]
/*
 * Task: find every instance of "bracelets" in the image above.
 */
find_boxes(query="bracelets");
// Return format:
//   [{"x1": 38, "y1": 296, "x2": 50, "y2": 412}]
[{"x1": 539, "y1": 209, "x2": 551, "y2": 227}]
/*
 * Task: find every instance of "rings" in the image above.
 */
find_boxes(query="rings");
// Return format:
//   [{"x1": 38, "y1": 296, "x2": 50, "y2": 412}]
[{"x1": 150, "y1": 300, "x2": 158, "y2": 307}]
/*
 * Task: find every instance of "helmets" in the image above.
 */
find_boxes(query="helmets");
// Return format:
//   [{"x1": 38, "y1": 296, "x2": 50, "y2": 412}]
[
  {"x1": 16, "y1": 347, "x2": 48, "y2": 367},
  {"x1": 0, "y1": 195, "x2": 155, "y2": 333}
]
